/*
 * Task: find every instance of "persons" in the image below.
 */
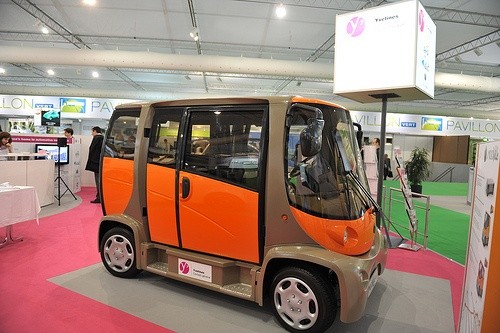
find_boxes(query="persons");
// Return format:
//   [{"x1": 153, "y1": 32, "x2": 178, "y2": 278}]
[
  {"x1": 64, "y1": 128, "x2": 75, "y2": 145},
  {"x1": 164, "y1": 139, "x2": 170, "y2": 153},
  {"x1": 383, "y1": 155, "x2": 391, "y2": 180},
  {"x1": 85, "y1": 126, "x2": 104, "y2": 203},
  {"x1": 115, "y1": 132, "x2": 124, "y2": 141},
  {"x1": 0, "y1": 132, "x2": 15, "y2": 161},
  {"x1": 294, "y1": 144, "x2": 302, "y2": 167},
  {"x1": 373, "y1": 138, "x2": 380, "y2": 180},
  {"x1": 128, "y1": 133, "x2": 136, "y2": 142}
]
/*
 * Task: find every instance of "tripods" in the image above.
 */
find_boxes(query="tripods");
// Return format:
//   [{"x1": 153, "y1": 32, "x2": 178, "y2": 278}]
[{"x1": 54, "y1": 147, "x2": 77, "y2": 206}]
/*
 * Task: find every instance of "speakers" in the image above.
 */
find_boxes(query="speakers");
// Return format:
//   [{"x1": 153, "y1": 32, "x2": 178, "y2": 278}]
[{"x1": 57, "y1": 138, "x2": 67, "y2": 147}]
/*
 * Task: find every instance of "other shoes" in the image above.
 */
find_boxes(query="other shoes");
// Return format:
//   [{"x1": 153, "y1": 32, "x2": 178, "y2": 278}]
[{"x1": 90, "y1": 199, "x2": 100, "y2": 203}]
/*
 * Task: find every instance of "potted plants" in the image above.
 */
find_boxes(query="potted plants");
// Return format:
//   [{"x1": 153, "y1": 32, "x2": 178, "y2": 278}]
[{"x1": 403, "y1": 147, "x2": 432, "y2": 198}]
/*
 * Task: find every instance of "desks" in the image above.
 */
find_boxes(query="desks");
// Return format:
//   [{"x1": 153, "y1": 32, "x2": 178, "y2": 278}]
[{"x1": 0, "y1": 183, "x2": 42, "y2": 247}]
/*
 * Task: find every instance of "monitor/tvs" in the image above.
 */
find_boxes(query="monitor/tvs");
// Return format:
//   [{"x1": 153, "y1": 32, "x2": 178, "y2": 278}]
[{"x1": 34, "y1": 144, "x2": 70, "y2": 165}]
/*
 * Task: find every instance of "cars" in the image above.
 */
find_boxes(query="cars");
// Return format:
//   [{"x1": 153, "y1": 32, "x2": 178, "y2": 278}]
[{"x1": 96, "y1": 95, "x2": 389, "y2": 333}]
